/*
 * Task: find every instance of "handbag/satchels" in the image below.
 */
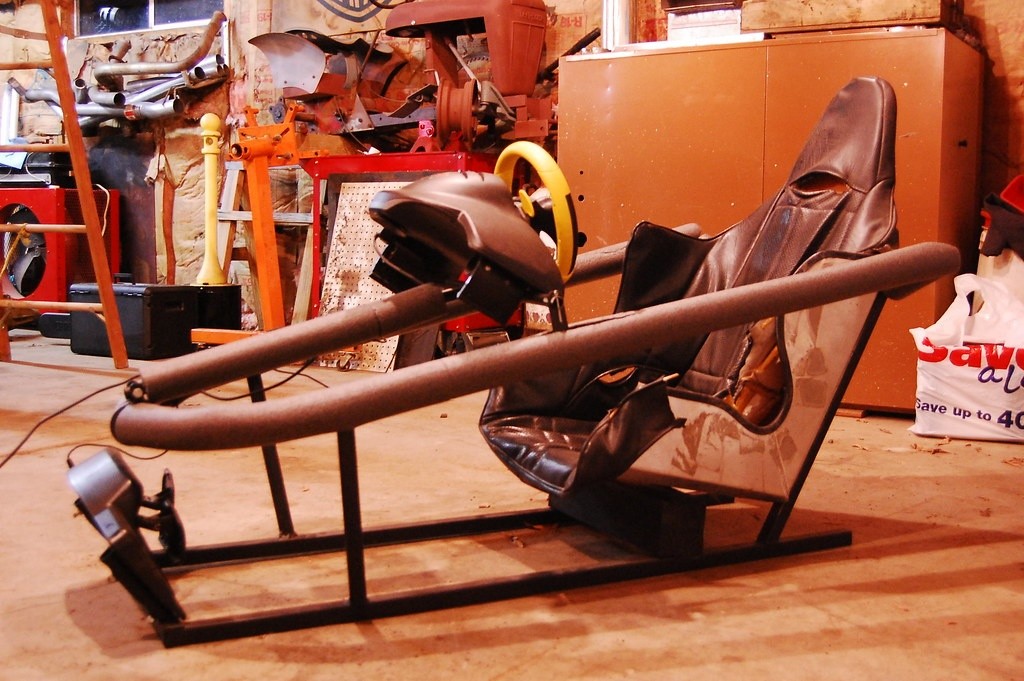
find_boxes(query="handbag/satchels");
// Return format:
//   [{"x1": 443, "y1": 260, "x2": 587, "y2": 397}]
[{"x1": 908, "y1": 272, "x2": 1024, "y2": 442}]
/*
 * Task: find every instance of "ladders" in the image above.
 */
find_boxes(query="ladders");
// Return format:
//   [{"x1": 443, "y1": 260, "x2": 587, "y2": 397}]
[{"x1": 0, "y1": 0, "x2": 130, "y2": 369}]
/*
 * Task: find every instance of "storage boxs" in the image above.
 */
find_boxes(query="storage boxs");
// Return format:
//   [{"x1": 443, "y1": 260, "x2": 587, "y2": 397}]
[
  {"x1": 68, "y1": 281, "x2": 246, "y2": 363},
  {"x1": 739, "y1": 0, "x2": 961, "y2": 36}
]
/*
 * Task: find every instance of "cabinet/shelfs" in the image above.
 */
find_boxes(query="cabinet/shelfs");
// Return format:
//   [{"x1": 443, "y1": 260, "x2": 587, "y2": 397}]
[{"x1": 557, "y1": 26, "x2": 989, "y2": 414}]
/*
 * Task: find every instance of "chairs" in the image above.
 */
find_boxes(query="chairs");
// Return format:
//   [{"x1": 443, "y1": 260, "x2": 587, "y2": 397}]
[{"x1": 467, "y1": 74, "x2": 901, "y2": 559}]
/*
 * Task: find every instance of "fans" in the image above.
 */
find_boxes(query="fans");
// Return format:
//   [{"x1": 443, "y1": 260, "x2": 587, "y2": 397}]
[{"x1": 0, "y1": 188, "x2": 120, "y2": 318}]
